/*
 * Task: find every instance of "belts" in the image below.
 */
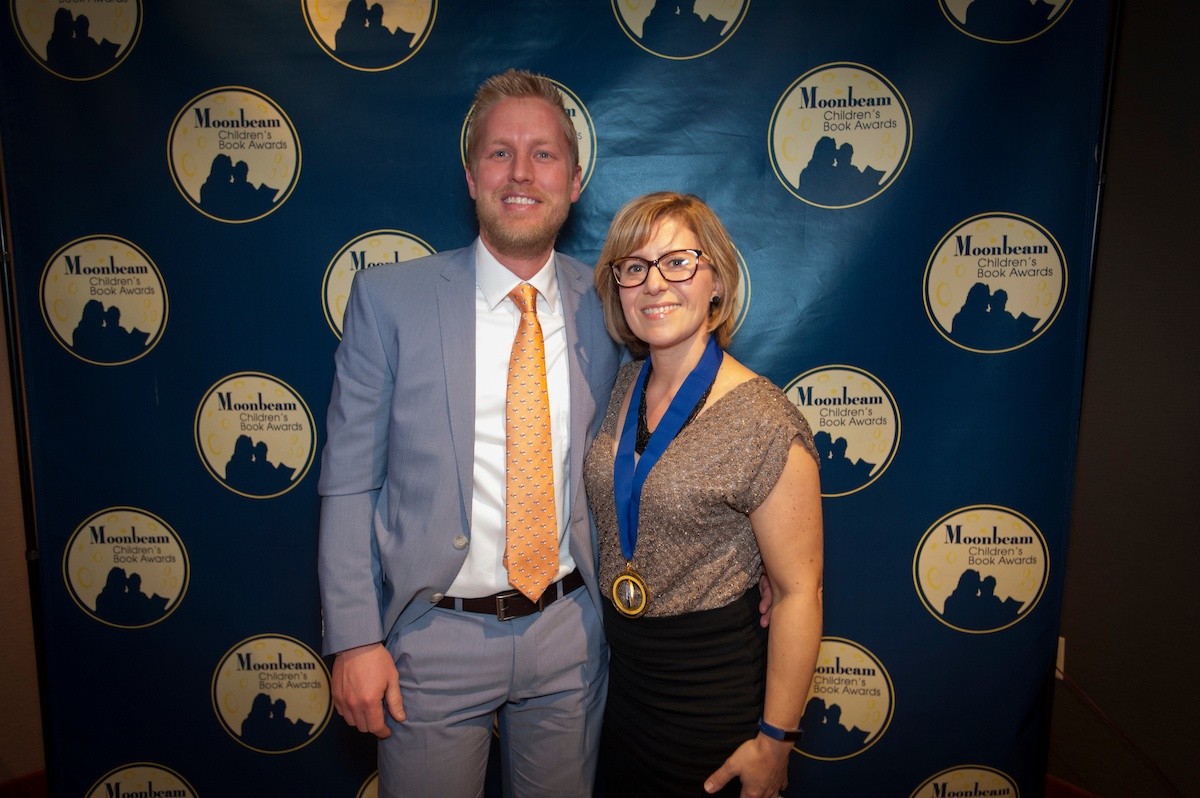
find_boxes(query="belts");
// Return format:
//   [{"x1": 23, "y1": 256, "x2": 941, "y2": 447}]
[{"x1": 435, "y1": 566, "x2": 584, "y2": 623}]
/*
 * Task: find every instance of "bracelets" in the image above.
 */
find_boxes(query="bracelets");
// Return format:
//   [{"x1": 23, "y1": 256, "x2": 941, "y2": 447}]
[{"x1": 758, "y1": 717, "x2": 804, "y2": 741}]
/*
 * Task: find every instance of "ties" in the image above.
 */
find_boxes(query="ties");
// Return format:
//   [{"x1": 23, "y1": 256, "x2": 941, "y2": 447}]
[{"x1": 505, "y1": 283, "x2": 560, "y2": 605}]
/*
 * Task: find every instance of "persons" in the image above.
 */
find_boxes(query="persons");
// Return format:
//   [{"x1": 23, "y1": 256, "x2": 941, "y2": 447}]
[
  {"x1": 582, "y1": 191, "x2": 824, "y2": 798},
  {"x1": 316, "y1": 71, "x2": 638, "y2": 798}
]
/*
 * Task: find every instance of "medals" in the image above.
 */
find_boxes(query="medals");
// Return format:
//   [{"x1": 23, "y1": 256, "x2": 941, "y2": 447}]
[{"x1": 610, "y1": 562, "x2": 651, "y2": 621}]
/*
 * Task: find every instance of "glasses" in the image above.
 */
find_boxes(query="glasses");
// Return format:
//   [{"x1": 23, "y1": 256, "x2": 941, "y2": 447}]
[{"x1": 609, "y1": 249, "x2": 718, "y2": 289}]
